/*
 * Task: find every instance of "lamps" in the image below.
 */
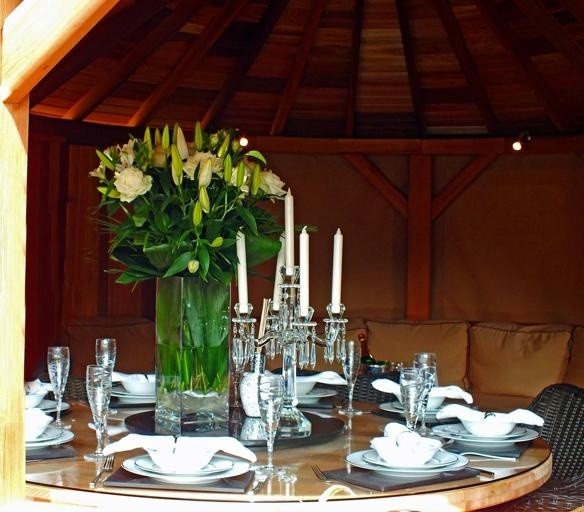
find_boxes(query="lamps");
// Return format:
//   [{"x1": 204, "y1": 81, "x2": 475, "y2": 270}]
[
  {"x1": 512, "y1": 132, "x2": 532, "y2": 156},
  {"x1": 234, "y1": 129, "x2": 250, "y2": 147}
]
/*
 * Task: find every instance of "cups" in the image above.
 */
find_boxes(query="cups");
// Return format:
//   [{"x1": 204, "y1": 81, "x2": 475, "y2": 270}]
[
  {"x1": 257, "y1": 375, "x2": 285, "y2": 476},
  {"x1": 399, "y1": 367, "x2": 425, "y2": 432},
  {"x1": 84, "y1": 365, "x2": 114, "y2": 461},
  {"x1": 340, "y1": 343, "x2": 362, "y2": 415},
  {"x1": 232, "y1": 339, "x2": 249, "y2": 373},
  {"x1": 47, "y1": 347, "x2": 72, "y2": 428},
  {"x1": 413, "y1": 352, "x2": 436, "y2": 435},
  {"x1": 96, "y1": 339, "x2": 117, "y2": 416}
]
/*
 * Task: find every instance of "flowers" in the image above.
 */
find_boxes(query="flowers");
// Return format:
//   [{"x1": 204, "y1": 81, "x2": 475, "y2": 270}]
[{"x1": 89, "y1": 120, "x2": 294, "y2": 276}]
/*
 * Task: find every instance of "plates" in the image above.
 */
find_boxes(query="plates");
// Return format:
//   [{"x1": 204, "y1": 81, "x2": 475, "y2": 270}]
[
  {"x1": 26, "y1": 427, "x2": 77, "y2": 450},
  {"x1": 296, "y1": 389, "x2": 337, "y2": 404},
  {"x1": 110, "y1": 393, "x2": 156, "y2": 403},
  {"x1": 41, "y1": 399, "x2": 57, "y2": 411},
  {"x1": 379, "y1": 402, "x2": 449, "y2": 418},
  {"x1": 25, "y1": 426, "x2": 63, "y2": 443},
  {"x1": 121, "y1": 454, "x2": 251, "y2": 484},
  {"x1": 42, "y1": 401, "x2": 70, "y2": 414},
  {"x1": 443, "y1": 423, "x2": 528, "y2": 440},
  {"x1": 431, "y1": 424, "x2": 539, "y2": 447},
  {"x1": 392, "y1": 401, "x2": 445, "y2": 412},
  {"x1": 112, "y1": 387, "x2": 156, "y2": 397},
  {"x1": 362, "y1": 449, "x2": 458, "y2": 471},
  {"x1": 344, "y1": 448, "x2": 470, "y2": 477},
  {"x1": 135, "y1": 456, "x2": 234, "y2": 477}
]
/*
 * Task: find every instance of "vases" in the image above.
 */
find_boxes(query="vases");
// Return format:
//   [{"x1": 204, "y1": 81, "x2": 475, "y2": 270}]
[{"x1": 154, "y1": 275, "x2": 228, "y2": 436}]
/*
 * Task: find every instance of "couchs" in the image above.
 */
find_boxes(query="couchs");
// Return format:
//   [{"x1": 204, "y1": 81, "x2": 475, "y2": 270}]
[{"x1": 64, "y1": 317, "x2": 581, "y2": 411}]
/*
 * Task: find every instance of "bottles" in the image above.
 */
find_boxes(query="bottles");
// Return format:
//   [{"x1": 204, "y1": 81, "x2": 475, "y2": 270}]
[{"x1": 359, "y1": 333, "x2": 377, "y2": 366}]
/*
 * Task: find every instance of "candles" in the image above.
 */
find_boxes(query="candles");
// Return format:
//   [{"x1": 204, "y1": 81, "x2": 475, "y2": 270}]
[
  {"x1": 235, "y1": 231, "x2": 249, "y2": 315},
  {"x1": 299, "y1": 228, "x2": 310, "y2": 317},
  {"x1": 284, "y1": 188, "x2": 294, "y2": 275},
  {"x1": 272, "y1": 238, "x2": 286, "y2": 311},
  {"x1": 331, "y1": 226, "x2": 344, "y2": 315}
]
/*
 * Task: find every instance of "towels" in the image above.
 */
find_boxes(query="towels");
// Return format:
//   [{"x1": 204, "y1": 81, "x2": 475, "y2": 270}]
[
  {"x1": 437, "y1": 403, "x2": 546, "y2": 426},
  {"x1": 111, "y1": 371, "x2": 158, "y2": 383},
  {"x1": 265, "y1": 367, "x2": 348, "y2": 385},
  {"x1": 23, "y1": 379, "x2": 66, "y2": 396},
  {"x1": 370, "y1": 423, "x2": 442, "y2": 451},
  {"x1": 25, "y1": 407, "x2": 46, "y2": 422},
  {"x1": 102, "y1": 432, "x2": 258, "y2": 464},
  {"x1": 371, "y1": 378, "x2": 474, "y2": 403}
]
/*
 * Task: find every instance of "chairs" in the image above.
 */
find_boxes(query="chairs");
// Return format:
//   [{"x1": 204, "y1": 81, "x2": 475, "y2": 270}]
[
  {"x1": 36, "y1": 366, "x2": 90, "y2": 400},
  {"x1": 519, "y1": 382, "x2": 584, "y2": 492},
  {"x1": 334, "y1": 373, "x2": 406, "y2": 406}
]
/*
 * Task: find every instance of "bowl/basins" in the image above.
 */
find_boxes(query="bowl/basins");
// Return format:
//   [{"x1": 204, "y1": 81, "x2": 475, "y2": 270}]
[
  {"x1": 121, "y1": 380, "x2": 155, "y2": 395},
  {"x1": 296, "y1": 381, "x2": 317, "y2": 395},
  {"x1": 373, "y1": 438, "x2": 444, "y2": 467},
  {"x1": 394, "y1": 392, "x2": 445, "y2": 409},
  {"x1": 24, "y1": 416, "x2": 54, "y2": 441},
  {"x1": 25, "y1": 394, "x2": 45, "y2": 409},
  {"x1": 457, "y1": 414, "x2": 516, "y2": 436},
  {"x1": 142, "y1": 445, "x2": 219, "y2": 472}
]
